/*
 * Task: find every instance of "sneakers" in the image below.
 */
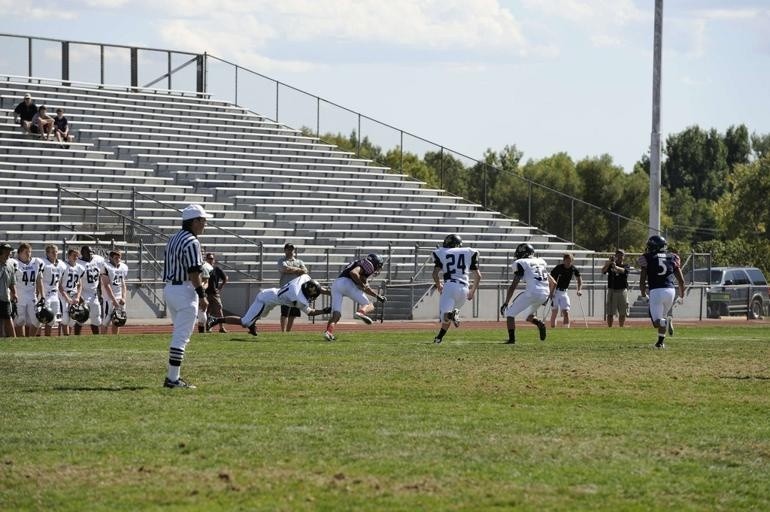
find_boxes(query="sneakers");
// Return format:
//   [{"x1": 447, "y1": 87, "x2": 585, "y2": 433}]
[
  {"x1": 163, "y1": 378, "x2": 196, "y2": 390},
  {"x1": 500, "y1": 340, "x2": 515, "y2": 344},
  {"x1": 648, "y1": 343, "x2": 665, "y2": 350},
  {"x1": 452, "y1": 309, "x2": 462, "y2": 328},
  {"x1": 199, "y1": 329, "x2": 228, "y2": 332},
  {"x1": 666, "y1": 316, "x2": 675, "y2": 337},
  {"x1": 539, "y1": 323, "x2": 546, "y2": 340},
  {"x1": 434, "y1": 337, "x2": 442, "y2": 345},
  {"x1": 324, "y1": 331, "x2": 336, "y2": 341},
  {"x1": 206, "y1": 316, "x2": 217, "y2": 330},
  {"x1": 356, "y1": 310, "x2": 373, "y2": 326},
  {"x1": 248, "y1": 326, "x2": 258, "y2": 336}
]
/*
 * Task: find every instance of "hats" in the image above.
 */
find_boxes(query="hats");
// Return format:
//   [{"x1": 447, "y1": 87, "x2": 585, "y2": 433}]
[
  {"x1": 23, "y1": 93, "x2": 31, "y2": 99},
  {"x1": 0, "y1": 243, "x2": 14, "y2": 252},
  {"x1": 182, "y1": 205, "x2": 214, "y2": 220},
  {"x1": 284, "y1": 243, "x2": 295, "y2": 249}
]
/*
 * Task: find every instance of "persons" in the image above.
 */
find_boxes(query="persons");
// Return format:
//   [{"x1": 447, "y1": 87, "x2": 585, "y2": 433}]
[
  {"x1": 549, "y1": 253, "x2": 586, "y2": 328},
  {"x1": 499, "y1": 242, "x2": 557, "y2": 344},
  {"x1": 276, "y1": 242, "x2": 308, "y2": 332},
  {"x1": 638, "y1": 233, "x2": 685, "y2": 349},
  {"x1": 323, "y1": 252, "x2": 388, "y2": 342},
  {"x1": 0, "y1": 241, "x2": 231, "y2": 337},
  {"x1": 160, "y1": 203, "x2": 215, "y2": 389},
  {"x1": 13, "y1": 92, "x2": 71, "y2": 148},
  {"x1": 205, "y1": 275, "x2": 332, "y2": 336},
  {"x1": 601, "y1": 248, "x2": 630, "y2": 327},
  {"x1": 429, "y1": 233, "x2": 483, "y2": 344}
]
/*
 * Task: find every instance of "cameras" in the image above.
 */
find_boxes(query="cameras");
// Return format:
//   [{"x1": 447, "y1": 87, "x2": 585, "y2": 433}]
[{"x1": 609, "y1": 254, "x2": 615, "y2": 262}]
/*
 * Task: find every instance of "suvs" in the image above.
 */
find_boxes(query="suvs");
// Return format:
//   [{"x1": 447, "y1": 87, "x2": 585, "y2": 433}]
[{"x1": 685, "y1": 265, "x2": 770, "y2": 320}]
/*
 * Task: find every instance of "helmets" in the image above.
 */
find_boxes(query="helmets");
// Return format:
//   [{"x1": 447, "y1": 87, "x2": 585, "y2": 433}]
[
  {"x1": 368, "y1": 253, "x2": 384, "y2": 271},
  {"x1": 443, "y1": 234, "x2": 463, "y2": 248},
  {"x1": 515, "y1": 244, "x2": 537, "y2": 258},
  {"x1": 110, "y1": 308, "x2": 128, "y2": 327},
  {"x1": 70, "y1": 300, "x2": 90, "y2": 322},
  {"x1": 35, "y1": 304, "x2": 54, "y2": 323},
  {"x1": 302, "y1": 279, "x2": 321, "y2": 301},
  {"x1": 648, "y1": 235, "x2": 670, "y2": 252}
]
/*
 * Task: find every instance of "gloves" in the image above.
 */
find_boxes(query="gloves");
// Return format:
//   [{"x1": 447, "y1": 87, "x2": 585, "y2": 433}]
[
  {"x1": 641, "y1": 294, "x2": 649, "y2": 303},
  {"x1": 674, "y1": 297, "x2": 683, "y2": 308},
  {"x1": 500, "y1": 302, "x2": 508, "y2": 317},
  {"x1": 376, "y1": 294, "x2": 387, "y2": 304},
  {"x1": 323, "y1": 306, "x2": 332, "y2": 314}
]
